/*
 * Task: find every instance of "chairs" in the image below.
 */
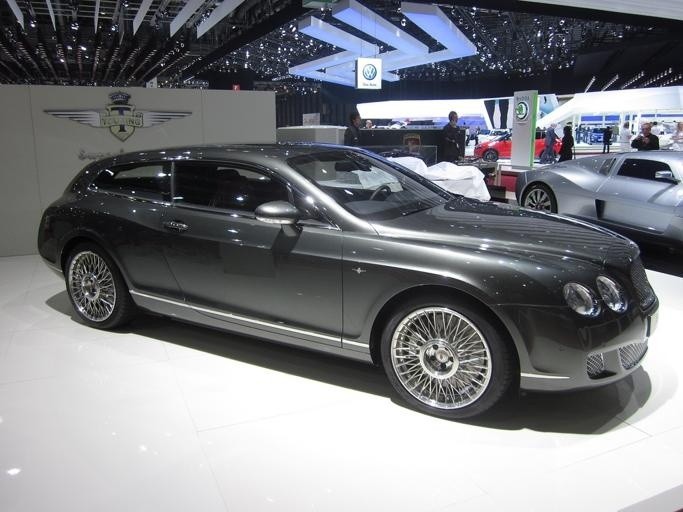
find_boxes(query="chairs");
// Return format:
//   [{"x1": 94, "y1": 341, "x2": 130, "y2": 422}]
[{"x1": 203, "y1": 176, "x2": 256, "y2": 211}]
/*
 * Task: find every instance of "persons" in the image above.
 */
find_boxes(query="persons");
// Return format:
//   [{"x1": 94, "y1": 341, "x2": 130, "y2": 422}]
[
  {"x1": 631, "y1": 123, "x2": 659, "y2": 151},
  {"x1": 618, "y1": 122, "x2": 633, "y2": 152},
  {"x1": 362, "y1": 120, "x2": 373, "y2": 129},
  {"x1": 438, "y1": 111, "x2": 461, "y2": 165},
  {"x1": 540, "y1": 122, "x2": 561, "y2": 164},
  {"x1": 602, "y1": 126, "x2": 612, "y2": 154},
  {"x1": 343, "y1": 112, "x2": 363, "y2": 148},
  {"x1": 556, "y1": 125, "x2": 574, "y2": 163}
]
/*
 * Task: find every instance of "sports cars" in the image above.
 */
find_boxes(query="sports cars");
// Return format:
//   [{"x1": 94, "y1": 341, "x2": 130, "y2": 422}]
[{"x1": 515, "y1": 151, "x2": 683, "y2": 250}]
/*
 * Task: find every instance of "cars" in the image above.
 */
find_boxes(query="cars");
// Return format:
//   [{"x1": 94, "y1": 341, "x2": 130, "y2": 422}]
[
  {"x1": 475, "y1": 126, "x2": 563, "y2": 163},
  {"x1": 37, "y1": 142, "x2": 659, "y2": 423},
  {"x1": 580, "y1": 126, "x2": 607, "y2": 145}
]
